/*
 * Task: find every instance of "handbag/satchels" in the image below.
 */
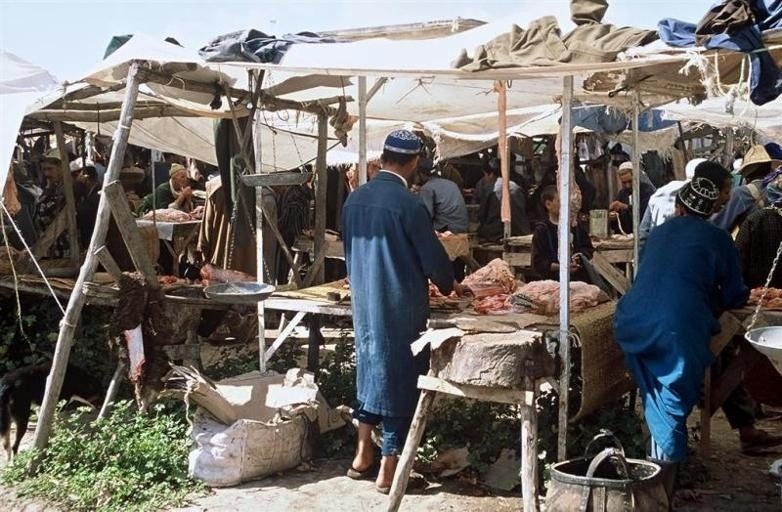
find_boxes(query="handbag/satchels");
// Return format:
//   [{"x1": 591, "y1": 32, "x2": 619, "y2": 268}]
[{"x1": 187, "y1": 370, "x2": 320, "y2": 488}]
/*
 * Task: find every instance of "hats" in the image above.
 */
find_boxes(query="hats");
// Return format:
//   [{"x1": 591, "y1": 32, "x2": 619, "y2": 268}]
[
  {"x1": 678, "y1": 178, "x2": 720, "y2": 215},
  {"x1": 384, "y1": 129, "x2": 420, "y2": 154},
  {"x1": 171, "y1": 164, "x2": 185, "y2": 175},
  {"x1": 42, "y1": 149, "x2": 95, "y2": 172},
  {"x1": 619, "y1": 162, "x2": 633, "y2": 170},
  {"x1": 736, "y1": 145, "x2": 782, "y2": 175}
]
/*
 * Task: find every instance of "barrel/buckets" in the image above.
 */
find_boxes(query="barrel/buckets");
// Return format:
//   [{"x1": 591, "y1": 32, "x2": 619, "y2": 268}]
[{"x1": 544, "y1": 432, "x2": 671, "y2": 512}]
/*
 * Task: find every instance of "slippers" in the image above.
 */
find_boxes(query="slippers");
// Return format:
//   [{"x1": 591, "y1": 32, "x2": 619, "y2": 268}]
[
  {"x1": 347, "y1": 447, "x2": 381, "y2": 479},
  {"x1": 376, "y1": 472, "x2": 426, "y2": 494}
]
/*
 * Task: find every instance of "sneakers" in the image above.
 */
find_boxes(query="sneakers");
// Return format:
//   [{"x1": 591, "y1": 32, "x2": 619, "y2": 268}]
[{"x1": 739, "y1": 430, "x2": 782, "y2": 452}]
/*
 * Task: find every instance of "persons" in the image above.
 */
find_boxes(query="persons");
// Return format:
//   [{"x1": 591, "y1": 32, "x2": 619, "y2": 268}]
[
  {"x1": 610, "y1": 178, "x2": 749, "y2": 499},
  {"x1": 1, "y1": 115, "x2": 781, "y2": 358},
  {"x1": 683, "y1": 162, "x2": 781, "y2": 456}
]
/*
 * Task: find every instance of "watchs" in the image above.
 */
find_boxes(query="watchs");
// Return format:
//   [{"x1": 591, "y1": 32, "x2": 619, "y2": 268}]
[{"x1": 338, "y1": 128, "x2": 473, "y2": 493}]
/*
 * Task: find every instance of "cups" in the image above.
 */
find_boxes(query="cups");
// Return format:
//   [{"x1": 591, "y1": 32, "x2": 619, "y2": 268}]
[{"x1": 588, "y1": 209, "x2": 607, "y2": 241}]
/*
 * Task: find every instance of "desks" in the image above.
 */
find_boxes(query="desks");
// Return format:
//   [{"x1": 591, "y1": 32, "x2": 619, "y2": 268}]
[
  {"x1": 255, "y1": 202, "x2": 781, "y2": 510},
  {"x1": 3, "y1": 201, "x2": 226, "y2": 418}
]
